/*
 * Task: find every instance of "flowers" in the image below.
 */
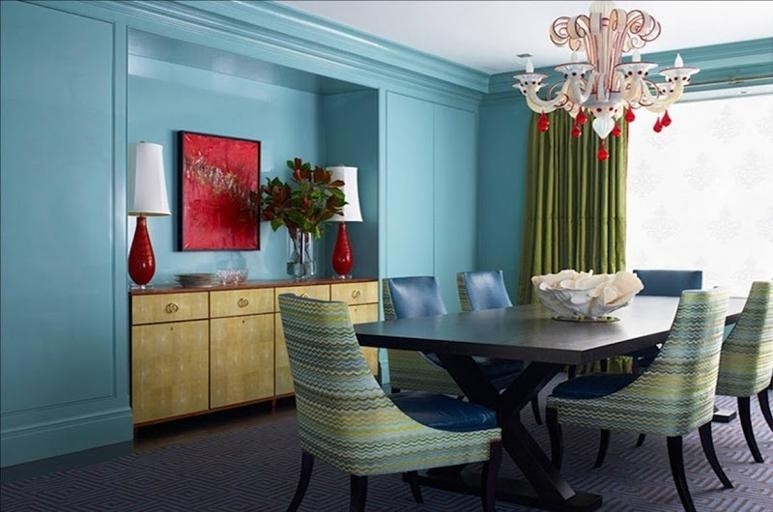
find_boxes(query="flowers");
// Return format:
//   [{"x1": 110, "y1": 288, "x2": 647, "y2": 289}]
[{"x1": 244, "y1": 158, "x2": 349, "y2": 261}]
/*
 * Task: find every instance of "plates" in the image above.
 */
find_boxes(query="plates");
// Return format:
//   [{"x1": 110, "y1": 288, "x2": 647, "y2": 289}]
[{"x1": 172, "y1": 272, "x2": 218, "y2": 289}]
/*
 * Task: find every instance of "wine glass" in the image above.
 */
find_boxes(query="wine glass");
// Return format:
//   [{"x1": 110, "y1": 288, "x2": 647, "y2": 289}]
[{"x1": 216, "y1": 258, "x2": 247, "y2": 287}]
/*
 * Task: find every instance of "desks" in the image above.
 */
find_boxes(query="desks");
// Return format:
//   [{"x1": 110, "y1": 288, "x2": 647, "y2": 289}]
[{"x1": 353, "y1": 295, "x2": 752, "y2": 512}]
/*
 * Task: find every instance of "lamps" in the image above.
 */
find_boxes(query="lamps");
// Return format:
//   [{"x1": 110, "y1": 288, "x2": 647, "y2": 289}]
[
  {"x1": 128, "y1": 140, "x2": 172, "y2": 290},
  {"x1": 512, "y1": 0, "x2": 701, "y2": 162},
  {"x1": 324, "y1": 167, "x2": 364, "y2": 279}
]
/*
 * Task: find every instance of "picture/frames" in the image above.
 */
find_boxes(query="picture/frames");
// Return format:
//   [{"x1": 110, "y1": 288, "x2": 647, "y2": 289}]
[{"x1": 177, "y1": 129, "x2": 262, "y2": 253}]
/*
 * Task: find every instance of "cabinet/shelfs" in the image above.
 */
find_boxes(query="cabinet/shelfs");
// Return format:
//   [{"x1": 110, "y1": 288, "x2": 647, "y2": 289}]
[
  {"x1": 274, "y1": 279, "x2": 380, "y2": 398},
  {"x1": 132, "y1": 282, "x2": 276, "y2": 426}
]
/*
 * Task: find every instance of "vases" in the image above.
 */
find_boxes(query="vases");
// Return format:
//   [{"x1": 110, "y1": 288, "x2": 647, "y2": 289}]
[{"x1": 287, "y1": 226, "x2": 317, "y2": 283}]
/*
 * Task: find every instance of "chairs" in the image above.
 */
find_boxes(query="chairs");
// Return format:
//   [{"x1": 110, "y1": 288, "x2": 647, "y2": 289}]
[
  {"x1": 279, "y1": 293, "x2": 503, "y2": 512},
  {"x1": 382, "y1": 275, "x2": 529, "y2": 478},
  {"x1": 544, "y1": 288, "x2": 733, "y2": 512},
  {"x1": 602, "y1": 270, "x2": 703, "y2": 373},
  {"x1": 456, "y1": 269, "x2": 572, "y2": 424},
  {"x1": 634, "y1": 281, "x2": 773, "y2": 462}
]
